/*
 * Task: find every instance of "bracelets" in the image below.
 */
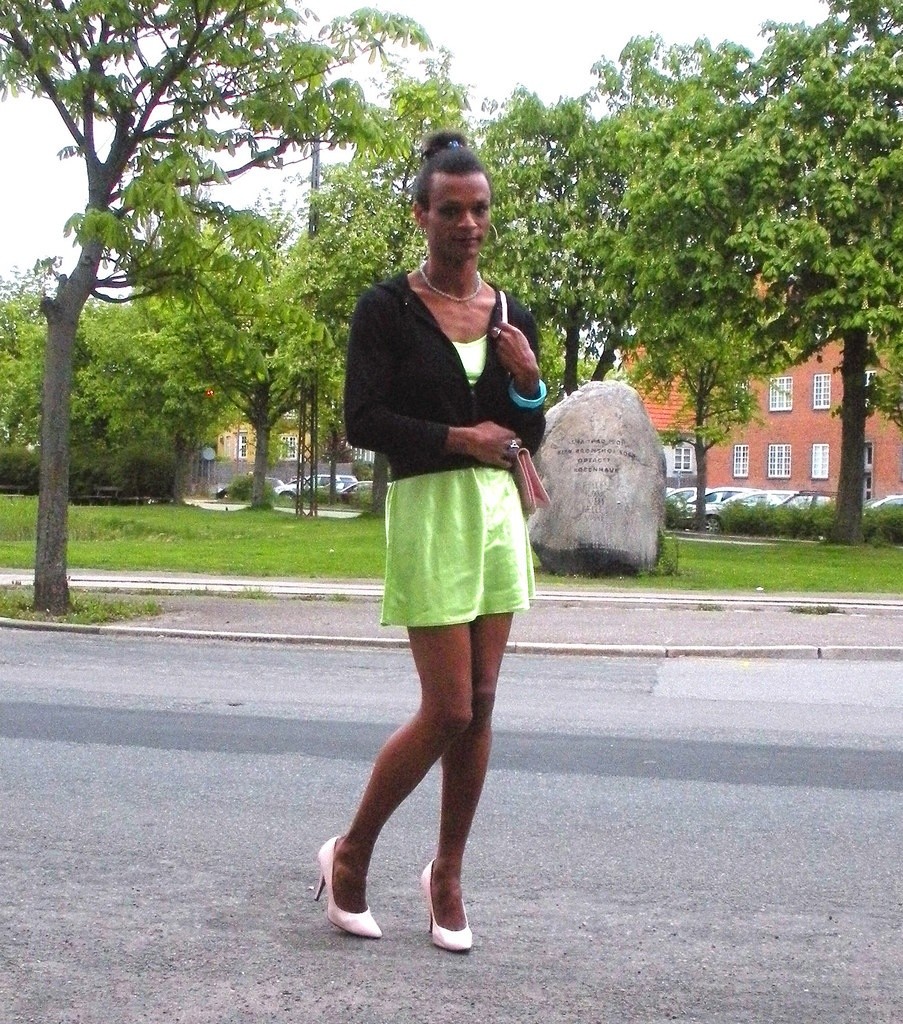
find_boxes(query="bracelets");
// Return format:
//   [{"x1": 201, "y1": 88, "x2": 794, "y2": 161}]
[{"x1": 508, "y1": 378, "x2": 547, "y2": 409}]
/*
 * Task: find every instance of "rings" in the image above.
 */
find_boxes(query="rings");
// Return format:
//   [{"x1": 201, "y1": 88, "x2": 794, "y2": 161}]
[
  {"x1": 509, "y1": 438, "x2": 519, "y2": 451},
  {"x1": 490, "y1": 327, "x2": 502, "y2": 340}
]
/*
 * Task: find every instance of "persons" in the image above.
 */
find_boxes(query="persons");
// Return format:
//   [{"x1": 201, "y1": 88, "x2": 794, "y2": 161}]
[{"x1": 311, "y1": 128, "x2": 548, "y2": 954}]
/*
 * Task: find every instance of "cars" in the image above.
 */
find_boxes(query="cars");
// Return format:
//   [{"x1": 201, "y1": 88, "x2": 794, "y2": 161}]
[
  {"x1": 864, "y1": 494, "x2": 903, "y2": 509},
  {"x1": 692, "y1": 490, "x2": 799, "y2": 534},
  {"x1": 665, "y1": 487, "x2": 712, "y2": 506},
  {"x1": 678, "y1": 488, "x2": 761, "y2": 509},
  {"x1": 265, "y1": 474, "x2": 372, "y2": 497},
  {"x1": 775, "y1": 490, "x2": 838, "y2": 509}
]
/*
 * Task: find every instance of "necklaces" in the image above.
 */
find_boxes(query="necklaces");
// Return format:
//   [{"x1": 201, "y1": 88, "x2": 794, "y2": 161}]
[{"x1": 420, "y1": 262, "x2": 482, "y2": 301}]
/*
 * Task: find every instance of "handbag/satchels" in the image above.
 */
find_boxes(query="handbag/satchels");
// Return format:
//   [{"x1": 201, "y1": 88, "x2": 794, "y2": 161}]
[{"x1": 512, "y1": 448, "x2": 553, "y2": 518}]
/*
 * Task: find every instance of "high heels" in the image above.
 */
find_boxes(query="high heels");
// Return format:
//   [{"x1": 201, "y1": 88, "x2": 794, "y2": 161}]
[
  {"x1": 421, "y1": 856, "x2": 473, "y2": 951},
  {"x1": 313, "y1": 836, "x2": 384, "y2": 939}
]
